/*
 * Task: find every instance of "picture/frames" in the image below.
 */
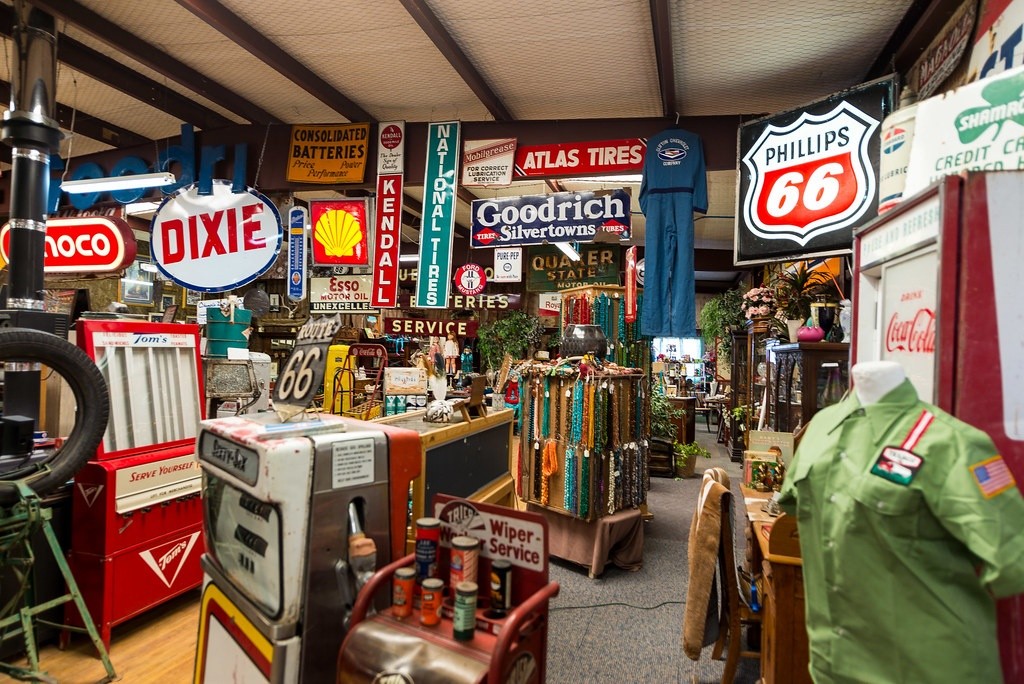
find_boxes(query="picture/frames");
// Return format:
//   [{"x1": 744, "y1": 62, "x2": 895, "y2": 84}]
[
  {"x1": 182, "y1": 287, "x2": 206, "y2": 310},
  {"x1": 186, "y1": 316, "x2": 204, "y2": 328},
  {"x1": 268, "y1": 293, "x2": 281, "y2": 313},
  {"x1": 117, "y1": 254, "x2": 156, "y2": 308},
  {"x1": 149, "y1": 312, "x2": 164, "y2": 323},
  {"x1": 159, "y1": 294, "x2": 175, "y2": 312}
]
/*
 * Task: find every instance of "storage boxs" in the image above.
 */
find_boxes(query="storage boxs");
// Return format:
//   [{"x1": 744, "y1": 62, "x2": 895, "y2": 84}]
[
  {"x1": 397, "y1": 409, "x2": 406, "y2": 414},
  {"x1": 344, "y1": 399, "x2": 384, "y2": 421},
  {"x1": 386, "y1": 410, "x2": 395, "y2": 417},
  {"x1": 386, "y1": 395, "x2": 396, "y2": 411},
  {"x1": 407, "y1": 409, "x2": 416, "y2": 412},
  {"x1": 408, "y1": 395, "x2": 416, "y2": 410},
  {"x1": 397, "y1": 395, "x2": 407, "y2": 410},
  {"x1": 417, "y1": 395, "x2": 427, "y2": 410}
]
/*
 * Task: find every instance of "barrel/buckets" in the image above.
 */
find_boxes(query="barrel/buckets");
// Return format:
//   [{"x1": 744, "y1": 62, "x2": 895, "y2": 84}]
[{"x1": 207, "y1": 307, "x2": 252, "y2": 356}]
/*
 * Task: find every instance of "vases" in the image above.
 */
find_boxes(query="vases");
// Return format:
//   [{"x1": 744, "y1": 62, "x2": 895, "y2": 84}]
[
  {"x1": 797, "y1": 326, "x2": 825, "y2": 342},
  {"x1": 558, "y1": 324, "x2": 608, "y2": 364}
]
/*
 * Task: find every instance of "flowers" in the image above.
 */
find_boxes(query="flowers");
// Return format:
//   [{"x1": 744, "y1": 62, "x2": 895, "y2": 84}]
[{"x1": 741, "y1": 283, "x2": 777, "y2": 319}]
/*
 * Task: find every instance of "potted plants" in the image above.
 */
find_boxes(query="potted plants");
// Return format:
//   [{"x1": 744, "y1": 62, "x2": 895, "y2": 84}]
[
  {"x1": 476, "y1": 311, "x2": 546, "y2": 409},
  {"x1": 758, "y1": 261, "x2": 840, "y2": 344},
  {"x1": 673, "y1": 439, "x2": 713, "y2": 479},
  {"x1": 650, "y1": 381, "x2": 688, "y2": 477},
  {"x1": 699, "y1": 280, "x2": 755, "y2": 365}
]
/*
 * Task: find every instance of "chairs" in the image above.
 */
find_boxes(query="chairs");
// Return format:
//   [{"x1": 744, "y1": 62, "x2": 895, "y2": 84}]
[{"x1": 704, "y1": 467, "x2": 761, "y2": 684}]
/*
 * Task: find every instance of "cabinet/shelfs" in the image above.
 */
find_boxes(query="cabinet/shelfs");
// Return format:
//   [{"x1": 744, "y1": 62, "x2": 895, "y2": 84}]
[{"x1": 367, "y1": 327, "x2": 850, "y2": 684}]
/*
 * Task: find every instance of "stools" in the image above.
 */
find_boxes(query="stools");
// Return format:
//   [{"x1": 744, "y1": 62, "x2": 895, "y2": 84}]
[{"x1": 695, "y1": 407, "x2": 712, "y2": 434}]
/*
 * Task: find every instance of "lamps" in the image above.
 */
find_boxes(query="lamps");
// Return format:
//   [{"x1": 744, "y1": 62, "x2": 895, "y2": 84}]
[
  {"x1": 555, "y1": 243, "x2": 582, "y2": 262},
  {"x1": 52, "y1": 42, "x2": 177, "y2": 194}
]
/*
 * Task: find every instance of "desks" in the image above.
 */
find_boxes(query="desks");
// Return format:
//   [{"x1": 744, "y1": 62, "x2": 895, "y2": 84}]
[
  {"x1": 705, "y1": 399, "x2": 730, "y2": 434},
  {"x1": 354, "y1": 378, "x2": 374, "y2": 394}
]
